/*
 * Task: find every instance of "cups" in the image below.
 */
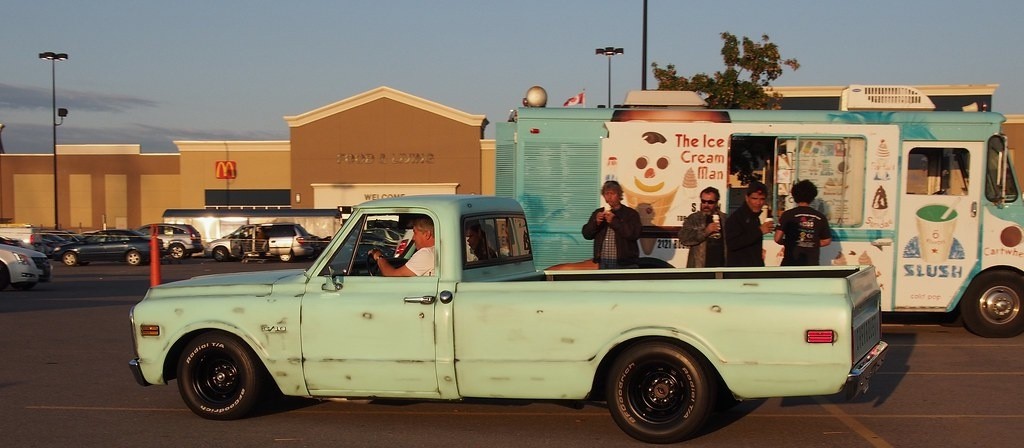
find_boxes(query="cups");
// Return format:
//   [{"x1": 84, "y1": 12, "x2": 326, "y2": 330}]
[{"x1": 915, "y1": 203, "x2": 961, "y2": 264}]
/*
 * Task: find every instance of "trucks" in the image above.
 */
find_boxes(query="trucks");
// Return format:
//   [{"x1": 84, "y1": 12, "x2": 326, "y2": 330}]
[{"x1": 494, "y1": 84, "x2": 1024, "y2": 339}]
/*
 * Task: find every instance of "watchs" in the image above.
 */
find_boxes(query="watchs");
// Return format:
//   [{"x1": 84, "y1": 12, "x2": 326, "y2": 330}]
[{"x1": 375, "y1": 255, "x2": 386, "y2": 261}]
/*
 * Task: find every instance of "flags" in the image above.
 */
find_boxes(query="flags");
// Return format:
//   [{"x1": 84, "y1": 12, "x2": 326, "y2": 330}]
[{"x1": 563, "y1": 92, "x2": 585, "y2": 107}]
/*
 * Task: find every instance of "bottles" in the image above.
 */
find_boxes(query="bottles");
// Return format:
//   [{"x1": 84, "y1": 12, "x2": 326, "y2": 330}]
[{"x1": 765, "y1": 216, "x2": 775, "y2": 231}]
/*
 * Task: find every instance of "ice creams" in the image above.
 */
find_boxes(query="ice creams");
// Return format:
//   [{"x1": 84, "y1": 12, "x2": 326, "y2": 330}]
[
  {"x1": 605, "y1": 202, "x2": 611, "y2": 212},
  {"x1": 712, "y1": 215, "x2": 720, "y2": 222}
]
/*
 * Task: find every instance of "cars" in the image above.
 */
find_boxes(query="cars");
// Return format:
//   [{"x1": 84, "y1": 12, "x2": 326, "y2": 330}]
[
  {"x1": 203, "y1": 222, "x2": 314, "y2": 263},
  {"x1": 0, "y1": 224, "x2": 86, "y2": 258},
  {"x1": 0, "y1": 244, "x2": 50, "y2": 292},
  {"x1": 51, "y1": 229, "x2": 165, "y2": 267},
  {"x1": 357, "y1": 227, "x2": 478, "y2": 262}
]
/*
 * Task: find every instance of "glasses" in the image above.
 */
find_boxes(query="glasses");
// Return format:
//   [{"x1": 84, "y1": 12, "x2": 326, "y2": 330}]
[{"x1": 702, "y1": 199, "x2": 717, "y2": 205}]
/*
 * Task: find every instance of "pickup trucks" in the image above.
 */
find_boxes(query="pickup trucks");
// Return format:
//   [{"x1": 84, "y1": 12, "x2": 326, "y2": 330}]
[{"x1": 128, "y1": 192, "x2": 891, "y2": 446}]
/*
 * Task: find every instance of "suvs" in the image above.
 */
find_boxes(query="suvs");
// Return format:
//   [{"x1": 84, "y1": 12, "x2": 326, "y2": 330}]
[{"x1": 134, "y1": 223, "x2": 204, "y2": 262}]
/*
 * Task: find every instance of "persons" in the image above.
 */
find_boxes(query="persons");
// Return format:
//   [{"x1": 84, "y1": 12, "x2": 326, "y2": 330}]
[
  {"x1": 582, "y1": 181, "x2": 641, "y2": 270},
  {"x1": 368, "y1": 217, "x2": 500, "y2": 277},
  {"x1": 725, "y1": 184, "x2": 775, "y2": 267},
  {"x1": 774, "y1": 179, "x2": 832, "y2": 266},
  {"x1": 679, "y1": 187, "x2": 728, "y2": 268},
  {"x1": 241, "y1": 226, "x2": 265, "y2": 263}
]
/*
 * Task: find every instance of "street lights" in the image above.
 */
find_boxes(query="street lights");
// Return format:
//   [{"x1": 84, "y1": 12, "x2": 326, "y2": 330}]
[
  {"x1": 38, "y1": 51, "x2": 68, "y2": 228},
  {"x1": 594, "y1": 46, "x2": 625, "y2": 108}
]
